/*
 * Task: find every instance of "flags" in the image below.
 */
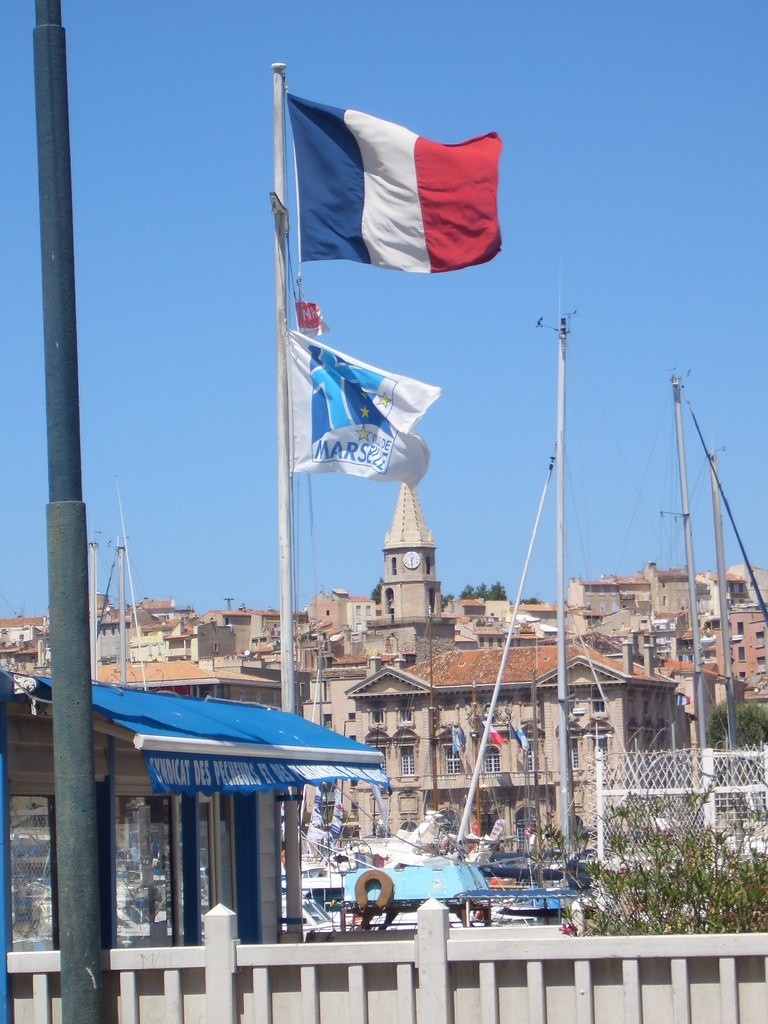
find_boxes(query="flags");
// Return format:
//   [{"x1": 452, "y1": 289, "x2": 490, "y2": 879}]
[
  {"x1": 305, "y1": 787, "x2": 344, "y2": 854},
  {"x1": 285, "y1": 328, "x2": 442, "y2": 492},
  {"x1": 451, "y1": 712, "x2": 530, "y2": 753},
  {"x1": 295, "y1": 301, "x2": 331, "y2": 338},
  {"x1": 285, "y1": 92, "x2": 502, "y2": 275},
  {"x1": 677, "y1": 693, "x2": 691, "y2": 706}
]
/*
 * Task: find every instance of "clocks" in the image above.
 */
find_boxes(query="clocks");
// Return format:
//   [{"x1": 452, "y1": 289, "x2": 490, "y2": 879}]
[{"x1": 403, "y1": 551, "x2": 420, "y2": 569}]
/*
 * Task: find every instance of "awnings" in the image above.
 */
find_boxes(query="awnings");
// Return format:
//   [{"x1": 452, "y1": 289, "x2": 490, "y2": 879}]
[{"x1": 25, "y1": 676, "x2": 393, "y2": 797}]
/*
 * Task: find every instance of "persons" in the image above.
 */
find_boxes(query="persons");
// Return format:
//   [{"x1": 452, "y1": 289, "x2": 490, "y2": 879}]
[{"x1": 525, "y1": 826, "x2": 545, "y2": 853}]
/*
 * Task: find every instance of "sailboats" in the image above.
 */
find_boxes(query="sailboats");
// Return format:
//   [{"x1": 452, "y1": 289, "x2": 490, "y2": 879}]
[{"x1": 88, "y1": 314, "x2": 767, "y2": 928}]
